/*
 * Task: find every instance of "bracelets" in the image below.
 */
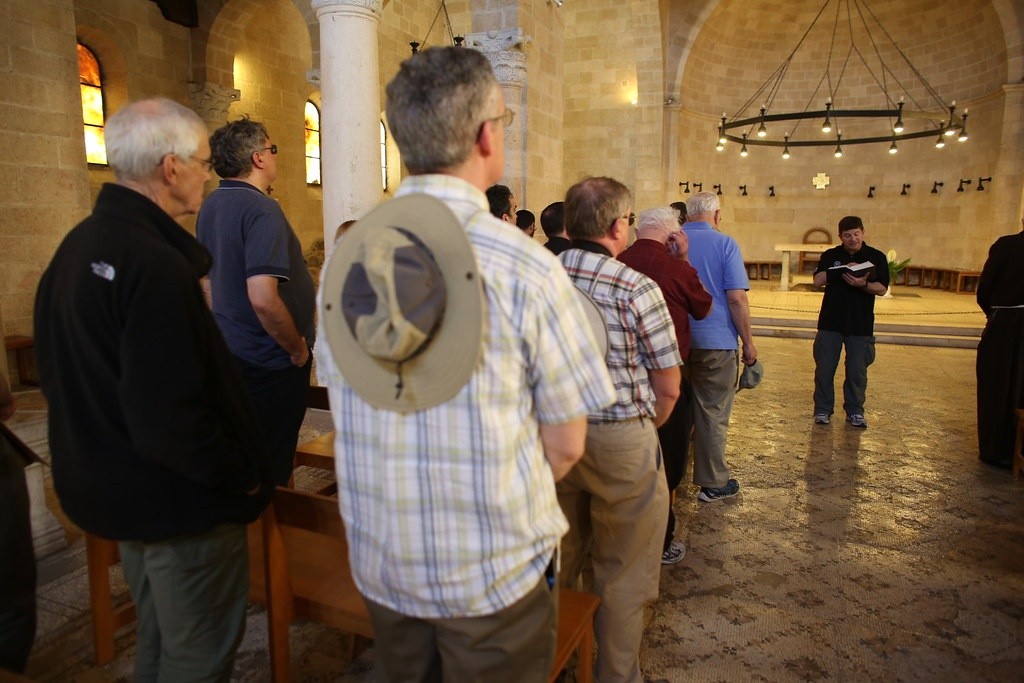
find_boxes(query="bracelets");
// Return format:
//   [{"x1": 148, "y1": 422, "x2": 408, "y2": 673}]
[{"x1": 861, "y1": 281, "x2": 868, "y2": 289}]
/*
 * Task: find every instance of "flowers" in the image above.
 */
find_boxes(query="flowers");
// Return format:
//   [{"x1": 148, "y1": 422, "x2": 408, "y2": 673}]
[{"x1": 884, "y1": 247, "x2": 911, "y2": 287}]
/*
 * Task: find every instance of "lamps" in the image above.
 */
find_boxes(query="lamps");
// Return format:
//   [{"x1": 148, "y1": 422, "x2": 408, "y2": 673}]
[
  {"x1": 693, "y1": 182, "x2": 705, "y2": 194},
  {"x1": 679, "y1": 179, "x2": 691, "y2": 195},
  {"x1": 713, "y1": 0, "x2": 970, "y2": 161},
  {"x1": 899, "y1": 183, "x2": 912, "y2": 196},
  {"x1": 977, "y1": 176, "x2": 992, "y2": 193},
  {"x1": 768, "y1": 184, "x2": 776, "y2": 197},
  {"x1": 956, "y1": 178, "x2": 972, "y2": 193},
  {"x1": 739, "y1": 184, "x2": 748, "y2": 196},
  {"x1": 713, "y1": 183, "x2": 723, "y2": 195},
  {"x1": 929, "y1": 181, "x2": 944, "y2": 194},
  {"x1": 867, "y1": 186, "x2": 876, "y2": 198}
]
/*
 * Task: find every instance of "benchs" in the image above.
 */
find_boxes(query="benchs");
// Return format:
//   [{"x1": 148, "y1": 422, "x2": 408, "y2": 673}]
[
  {"x1": 736, "y1": 259, "x2": 982, "y2": 298},
  {"x1": 84, "y1": 385, "x2": 607, "y2": 683}
]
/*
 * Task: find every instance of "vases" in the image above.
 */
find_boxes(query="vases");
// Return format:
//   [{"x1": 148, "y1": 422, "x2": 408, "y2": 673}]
[{"x1": 880, "y1": 286, "x2": 893, "y2": 298}]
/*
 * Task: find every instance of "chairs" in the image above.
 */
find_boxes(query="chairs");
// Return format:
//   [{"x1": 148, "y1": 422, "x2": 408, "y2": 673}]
[{"x1": 797, "y1": 227, "x2": 833, "y2": 274}]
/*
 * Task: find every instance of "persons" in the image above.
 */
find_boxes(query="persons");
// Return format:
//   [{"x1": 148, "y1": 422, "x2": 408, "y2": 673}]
[
  {"x1": 616, "y1": 208, "x2": 713, "y2": 564},
  {"x1": 813, "y1": 216, "x2": 890, "y2": 427},
  {"x1": 334, "y1": 220, "x2": 357, "y2": 244},
  {"x1": 486, "y1": 183, "x2": 518, "y2": 226},
  {"x1": 314, "y1": 45, "x2": 617, "y2": 683},
  {"x1": 554, "y1": 177, "x2": 684, "y2": 683},
  {"x1": 540, "y1": 202, "x2": 572, "y2": 257},
  {"x1": 34, "y1": 96, "x2": 277, "y2": 682},
  {"x1": 675, "y1": 191, "x2": 757, "y2": 502},
  {"x1": 976, "y1": 219, "x2": 1024, "y2": 473},
  {"x1": 515, "y1": 210, "x2": 536, "y2": 238},
  {"x1": 669, "y1": 202, "x2": 689, "y2": 227},
  {"x1": 0, "y1": 365, "x2": 49, "y2": 682},
  {"x1": 195, "y1": 115, "x2": 315, "y2": 488}
]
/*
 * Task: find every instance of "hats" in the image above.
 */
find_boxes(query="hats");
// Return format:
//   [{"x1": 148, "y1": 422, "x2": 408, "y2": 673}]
[
  {"x1": 321, "y1": 192, "x2": 485, "y2": 413},
  {"x1": 736, "y1": 358, "x2": 764, "y2": 393}
]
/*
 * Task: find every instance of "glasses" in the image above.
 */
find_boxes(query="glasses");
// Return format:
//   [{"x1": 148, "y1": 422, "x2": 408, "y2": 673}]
[
  {"x1": 475, "y1": 108, "x2": 515, "y2": 144},
  {"x1": 715, "y1": 210, "x2": 721, "y2": 221},
  {"x1": 608, "y1": 211, "x2": 636, "y2": 229},
  {"x1": 530, "y1": 223, "x2": 537, "y2": 231},
  {"x1": 156, "y1": 152, "x2": 216, "y2": 172},
  {"x1": 250, "y1": 144, "x2": 277, "y2": 160}
]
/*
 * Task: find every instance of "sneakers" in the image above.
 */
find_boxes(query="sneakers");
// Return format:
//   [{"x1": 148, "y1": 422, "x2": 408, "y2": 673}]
[
  {"x1": 660, "y1": 542, "x2": 687, "y2": 564},
  {"x1": 815, "y1": 414, "x2": 829, "y2": 424},
  {"x1": 698, "y1": 479, "x2": 739, "y2": 502},
  {"x1": 846, "y1": 413, "x2": 866, "y2": 427}
]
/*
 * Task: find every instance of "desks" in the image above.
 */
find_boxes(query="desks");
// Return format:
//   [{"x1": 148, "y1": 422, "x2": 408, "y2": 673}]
[{"x1": 775, "y1": 241, "x2": 842, "y2": 292}]
